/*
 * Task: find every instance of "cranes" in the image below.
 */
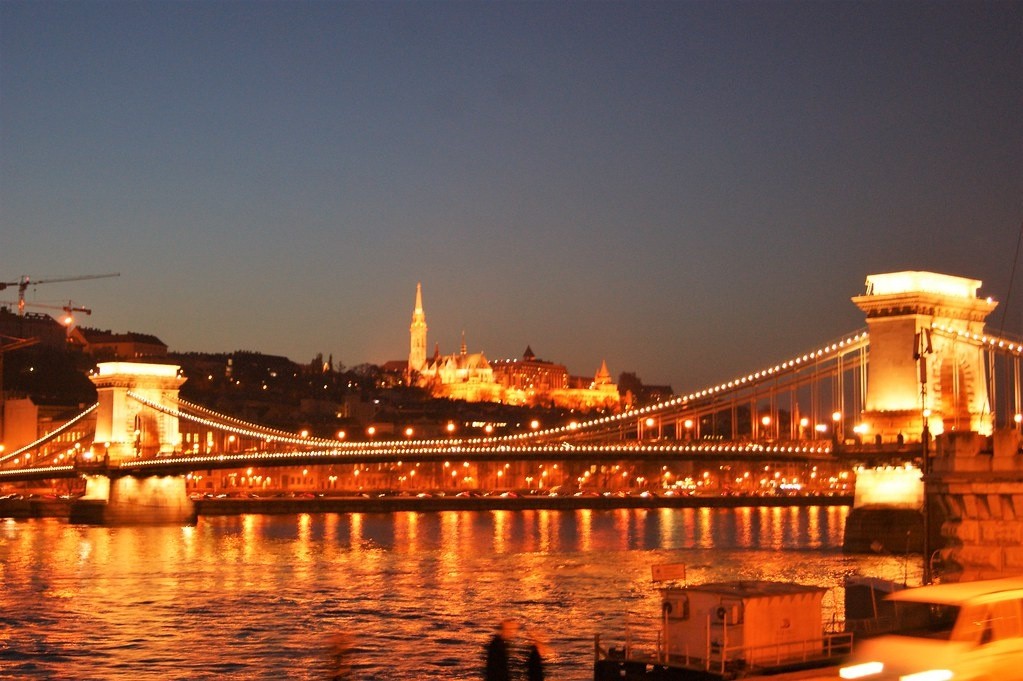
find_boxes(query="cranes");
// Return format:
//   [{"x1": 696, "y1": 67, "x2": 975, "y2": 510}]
[
  {"x1": 0, "y1": 298, "x2": 92, "y2": 334},
  {"x1": 0, "y1": 272, "x2": 121, "y2": 316}
]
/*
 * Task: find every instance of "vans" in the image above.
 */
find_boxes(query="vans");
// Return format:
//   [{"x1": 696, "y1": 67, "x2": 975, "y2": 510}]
[{"x1": 841, "y1": 577, "x2": 1023, "y2": 681}]
